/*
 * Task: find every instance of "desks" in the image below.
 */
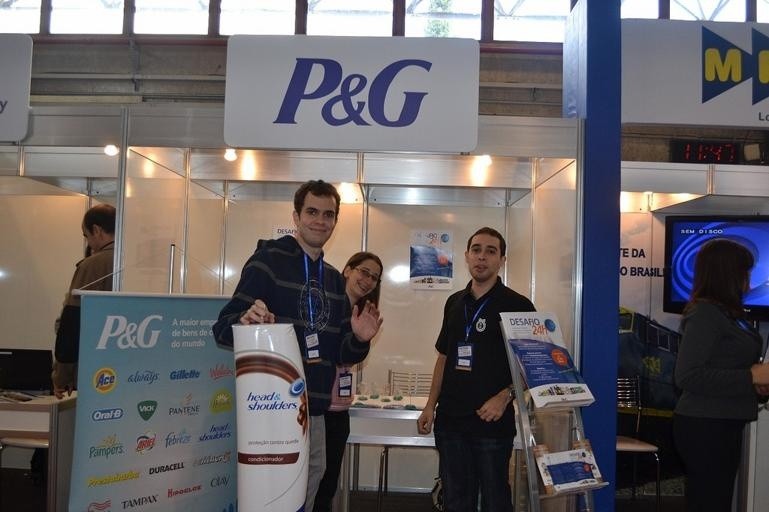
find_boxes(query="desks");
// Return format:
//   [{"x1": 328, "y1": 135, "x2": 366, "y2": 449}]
[
  {"x1": 0, "y1": 391, "x2": 76, "y2": 512},
  {"x1": 344, "y1": 393, "x2": 536, "y2": 511}
]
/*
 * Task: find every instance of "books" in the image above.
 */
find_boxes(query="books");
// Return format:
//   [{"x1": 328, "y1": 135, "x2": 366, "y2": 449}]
[{"x1": 499, "y1": 311, "x2": 597, "y2": 409}]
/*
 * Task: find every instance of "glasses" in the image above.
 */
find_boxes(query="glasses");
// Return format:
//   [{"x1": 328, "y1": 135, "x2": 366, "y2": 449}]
[{"x1": 354, "y1": 266, "x2": 380, "y2": 282}]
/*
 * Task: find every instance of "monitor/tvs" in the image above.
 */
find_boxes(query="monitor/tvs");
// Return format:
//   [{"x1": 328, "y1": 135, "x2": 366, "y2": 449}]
[{"x1": 663, "y1": 215, "x2": 769, "y2": 323}]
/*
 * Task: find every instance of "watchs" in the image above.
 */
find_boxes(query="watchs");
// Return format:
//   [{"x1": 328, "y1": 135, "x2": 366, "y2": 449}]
[{"x1": 507, "y1": 384, "x2": 516, "y2": 400}]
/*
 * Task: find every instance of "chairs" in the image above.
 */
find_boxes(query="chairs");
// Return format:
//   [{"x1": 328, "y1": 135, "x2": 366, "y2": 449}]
[{"x1": 377, "y1": 368, "x2": 435, "y2": 510}]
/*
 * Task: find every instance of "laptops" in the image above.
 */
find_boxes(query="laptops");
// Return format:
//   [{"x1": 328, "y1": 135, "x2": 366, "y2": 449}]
[{"x1": 0, "y1": 348, "x2": 53, "y2": 395}]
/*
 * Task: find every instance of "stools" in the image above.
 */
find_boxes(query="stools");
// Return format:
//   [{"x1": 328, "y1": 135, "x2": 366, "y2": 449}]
[{"x1": 616, "y1": 436, "x2": 661, "y2": 512}]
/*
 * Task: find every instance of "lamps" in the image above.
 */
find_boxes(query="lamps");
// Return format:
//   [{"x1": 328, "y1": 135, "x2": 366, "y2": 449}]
[{"x1": 224, "y1": 149, "x2": 237, "y2": 161}]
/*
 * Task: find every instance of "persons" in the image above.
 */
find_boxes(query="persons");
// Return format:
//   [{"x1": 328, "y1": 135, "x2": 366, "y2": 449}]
[
  {"x1": 672, "y1": 239, "x2": 769, "y2": 512},
  {"x1": 212, "y1": 180, "x2": 383, "y2": 512},
  {"x1": 311, "y1": 252, "x2": 383, "y2": 512},
  {"x1": 418, "y1": 228, "x2": 537, "y2": 512},
  {"x1": 52, "y1": 204, "x2": 115, "y2": 400}
]
